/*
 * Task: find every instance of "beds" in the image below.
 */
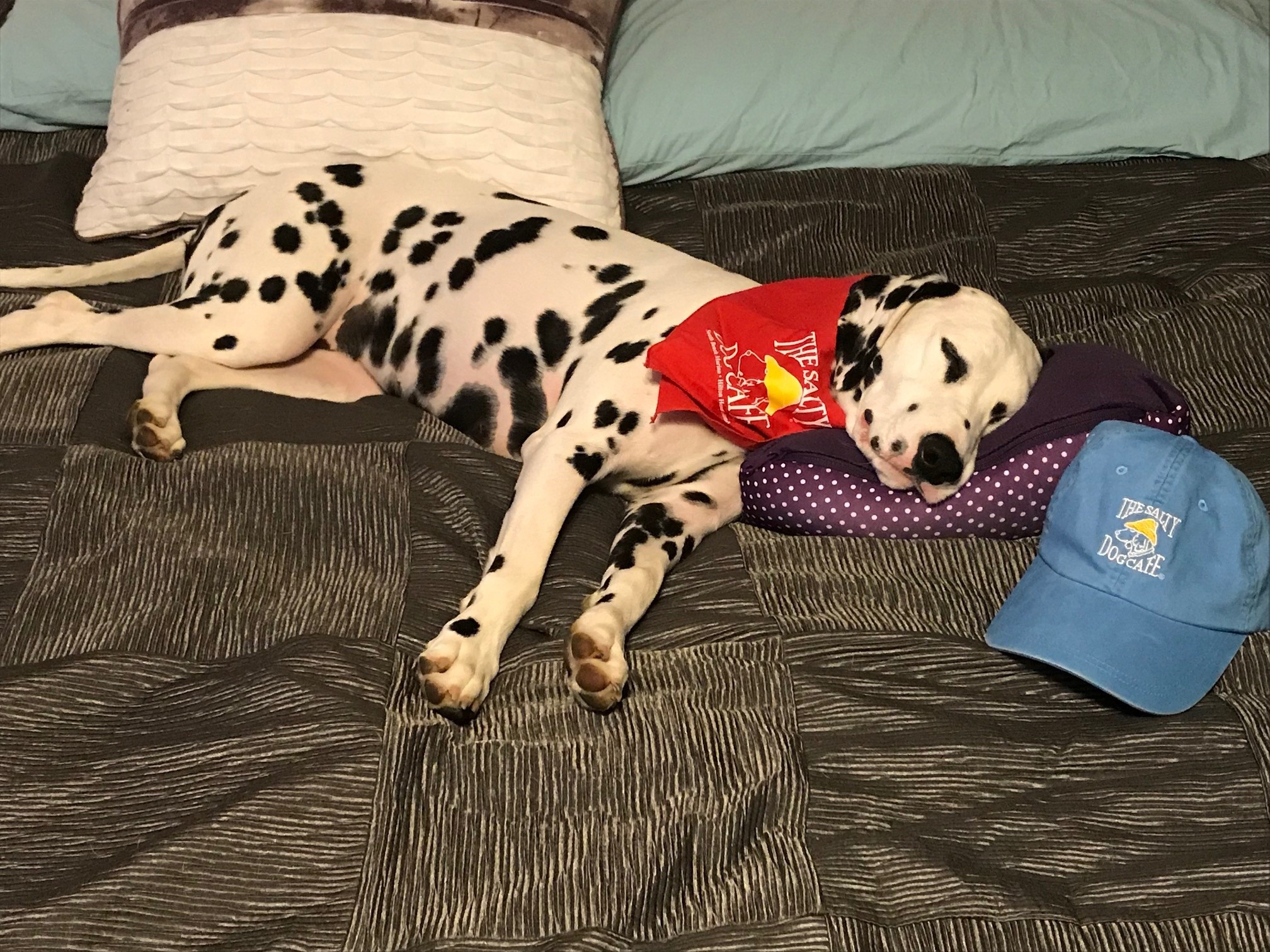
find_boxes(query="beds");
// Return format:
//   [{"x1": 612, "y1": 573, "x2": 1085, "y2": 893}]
[{"x1": 0, "y1": 0, "x2": 1270, "y2": 952}]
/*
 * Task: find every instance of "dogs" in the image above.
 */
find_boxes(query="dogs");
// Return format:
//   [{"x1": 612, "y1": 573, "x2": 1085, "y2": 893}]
[{"x1": 0, "y1": 163, "x2": 1044, "y2": 726}]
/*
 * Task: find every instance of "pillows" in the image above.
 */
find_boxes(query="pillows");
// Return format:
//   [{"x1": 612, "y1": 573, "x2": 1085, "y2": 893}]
[
  {"x1": 0, "y1": 0, "x2": 1270, "y2": 242},
  {"x1": 737, "y1": 343, "x2": 1192, "y2": 539}
]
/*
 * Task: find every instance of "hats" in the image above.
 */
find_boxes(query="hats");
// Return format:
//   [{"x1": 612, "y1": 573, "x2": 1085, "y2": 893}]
[{"x1": 982, "y1": 418, "x2": 1270, "y2": 718}]
